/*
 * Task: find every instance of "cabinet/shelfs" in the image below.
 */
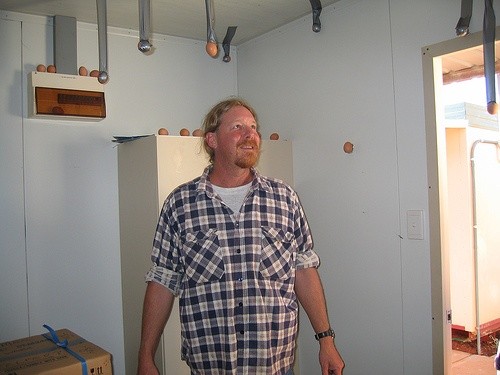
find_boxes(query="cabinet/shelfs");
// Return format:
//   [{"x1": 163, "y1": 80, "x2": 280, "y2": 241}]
[{"x1": 117, "y1": 134, "x2": 299, "y2": 375}]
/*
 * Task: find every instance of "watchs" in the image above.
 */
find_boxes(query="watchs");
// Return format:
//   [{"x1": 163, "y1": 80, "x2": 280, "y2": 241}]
[{"x1": 315, "y1": 329, "x2": 335, "y2": 340}]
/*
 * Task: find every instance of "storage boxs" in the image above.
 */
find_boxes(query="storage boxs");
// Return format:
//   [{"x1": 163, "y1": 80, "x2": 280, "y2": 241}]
[{"x1": 0, "y1": 328, "x2": 113, "y2": 375}]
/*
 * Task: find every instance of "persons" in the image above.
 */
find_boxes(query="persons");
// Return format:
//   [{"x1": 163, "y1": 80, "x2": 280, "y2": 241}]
[{"x1": 136, "y1": 99, "x2": 345, "y2": 375}]
